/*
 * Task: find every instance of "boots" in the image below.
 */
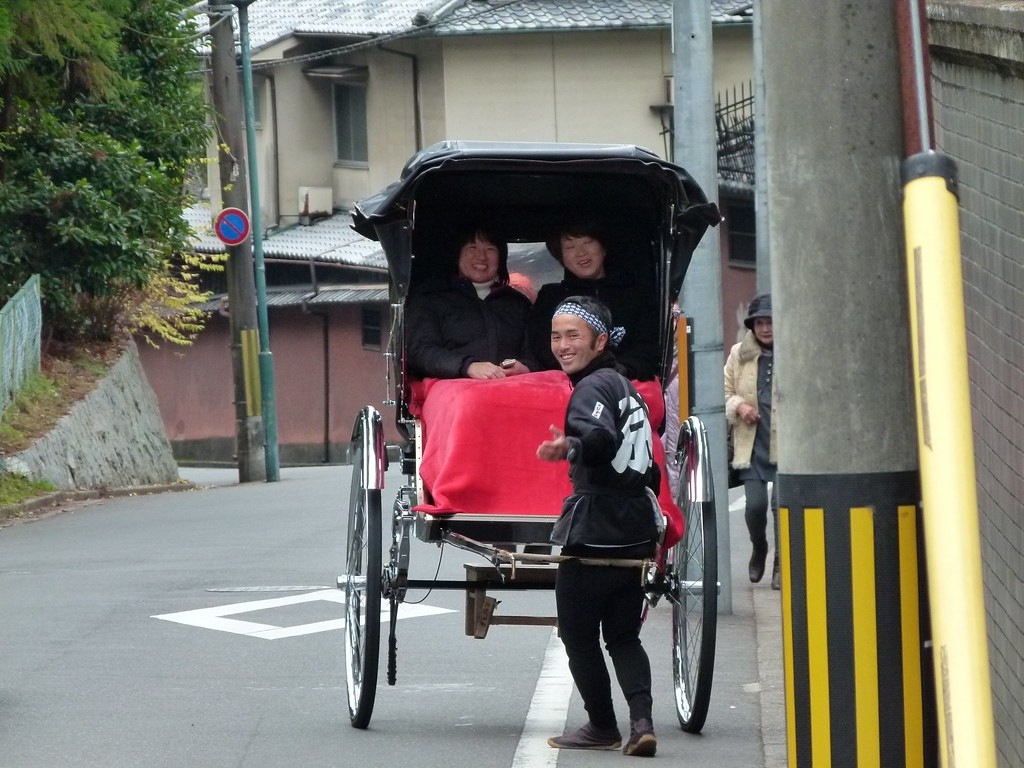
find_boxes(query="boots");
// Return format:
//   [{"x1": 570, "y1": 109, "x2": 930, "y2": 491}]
[
  {"x1": 771, "y1": 550, "x2": 781, "y2": 590},
  {"x1": 746, "y1": 520, "x2": 768, "y2": 583}
]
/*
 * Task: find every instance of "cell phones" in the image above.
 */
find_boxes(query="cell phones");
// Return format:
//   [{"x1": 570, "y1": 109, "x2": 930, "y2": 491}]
[{"x1": 500, "y1": 359, "x2": 516, "y2": 369}]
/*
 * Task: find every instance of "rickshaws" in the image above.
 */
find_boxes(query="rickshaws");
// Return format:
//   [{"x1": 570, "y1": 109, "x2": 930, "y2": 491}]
[{"x1": 336, "y1": 137, "x2": 724, "y2": 734}]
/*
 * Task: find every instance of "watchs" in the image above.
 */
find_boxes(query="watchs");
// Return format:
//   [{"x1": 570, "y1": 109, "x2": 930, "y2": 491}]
[{"x1": 565, "y1": 443, "x2": 577, "y2": 461}]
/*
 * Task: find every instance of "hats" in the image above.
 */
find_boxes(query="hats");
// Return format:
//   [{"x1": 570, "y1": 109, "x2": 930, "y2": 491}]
[{"x1": 744, "y1": 295, "x2": 772, "y2": 329}]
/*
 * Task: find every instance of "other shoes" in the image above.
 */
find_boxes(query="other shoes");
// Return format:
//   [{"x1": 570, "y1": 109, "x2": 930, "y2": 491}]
[
  {"x1": 547, "y1": 721, "x2": 622, "y2": 750},
  {"x1": 623, "y1": 717, "x2": 657, "y2": 755}
]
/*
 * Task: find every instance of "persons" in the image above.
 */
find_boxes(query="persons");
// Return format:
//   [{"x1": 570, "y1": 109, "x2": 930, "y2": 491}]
[
  {"x1": 724, "y1": 293, "x2": 781, "y2": 590},
  {"x1": 536, "y1": 296, "x2": 662, "y2": 757},
  {"x1": 406, "y1": 221, "x2": 533, "y2": 379},
  {"x1": 528, "y1": 217, "x2": 654, "y2": 376}
]
